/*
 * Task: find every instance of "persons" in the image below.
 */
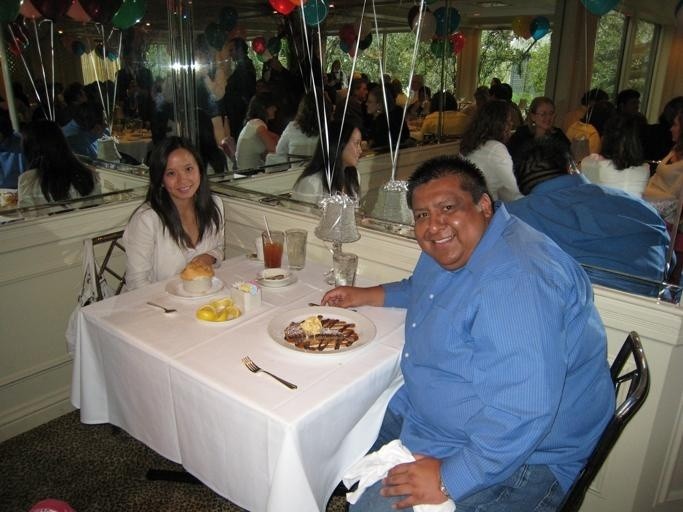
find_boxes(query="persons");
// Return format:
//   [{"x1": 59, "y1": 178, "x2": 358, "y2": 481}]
[
  {"x1": 123, "y1": 136, "x2": 226, "y2": 292},
  {"x1": 321, "y1": 154, "x2": 618, "y2": 512},
  {"x1": 1, "y1": 62, "x2": 154, "y2": 219},
  {"x1": 460, "y1": 77, "x2": 681, "y2": 299},
  {"x1": 196, "y1": 41, "x2": 458, "y2": 175}
]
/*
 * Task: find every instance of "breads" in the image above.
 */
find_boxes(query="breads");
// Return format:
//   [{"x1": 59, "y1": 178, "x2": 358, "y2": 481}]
[{"x1": 181, "y1": 262, "x2": 215, "y2": 294}]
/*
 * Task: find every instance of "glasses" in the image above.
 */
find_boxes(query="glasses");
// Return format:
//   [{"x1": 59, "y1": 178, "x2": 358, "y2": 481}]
[{"x1": 535, "y1": 110, "x2": 557, "y2": 118}]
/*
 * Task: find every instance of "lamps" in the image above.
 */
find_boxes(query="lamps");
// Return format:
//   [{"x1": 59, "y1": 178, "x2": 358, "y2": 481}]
[
  {"x1": 313, "y1": 198, "x2": 359, "y2": 288},
  {"x1": 96, "y1": 139, "x2": 122, "y2": 164},
  {"x1": 375, "y1": 182, "x2": 414, "y2": 227}
]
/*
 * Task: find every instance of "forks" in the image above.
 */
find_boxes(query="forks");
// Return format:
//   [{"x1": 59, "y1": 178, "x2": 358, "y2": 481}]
[{"x1": 240, "y1": 354, "x2": 300, "y2": 390}]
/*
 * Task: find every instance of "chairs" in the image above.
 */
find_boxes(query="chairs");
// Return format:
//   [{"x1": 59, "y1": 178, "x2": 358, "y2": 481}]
[
  {"x1": 221, "y1": 134, "x2": 238, "y2": 166},
  {"x1": 85, "y1": 230, "x2": 129, "y2": 302},
  {"x1": 558, "y1": 331, "x2": 650, "y2": 512}
]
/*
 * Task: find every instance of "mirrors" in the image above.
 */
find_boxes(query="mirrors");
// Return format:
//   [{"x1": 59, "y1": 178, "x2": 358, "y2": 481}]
[
  {"x1": 1, "y1": 0, "x2": 184, "y2": 228},
  {"x1": 178, "y1": 1, "x2": 682, "y2": 304}
]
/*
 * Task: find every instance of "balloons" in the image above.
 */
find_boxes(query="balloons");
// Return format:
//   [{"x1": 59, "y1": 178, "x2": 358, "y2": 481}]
[
  {"x1": 512, "y1": 16, "x2": 550, "y2": 40},
  {"x1": 270, "y1": 2, "x2": 328, "y2": 26},
  {"x1": 253, "y1": 33, "x2": 282, "y2": 60},
  {"x1": 1, "y1": 0, "x2": 147, "y2": 57},
  {"x1": 340, "y1": 18, "x2": 374, "y2": 58},
  {"x1": 197, "y1": 6, "x2": 250, "y2": 59},
  {"x1": 409, "y1": 7, "x2": 465, "y2": 58},
  {"x1": 580, "y1": 1, "x2": 618, "y2": 17}
]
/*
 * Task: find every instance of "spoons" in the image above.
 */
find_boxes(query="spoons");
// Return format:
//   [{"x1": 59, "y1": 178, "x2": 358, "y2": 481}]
[
  {"x1": 253, "y1": 275, "x2": 284, "y2": 282},
  {"x1": 145, "y1": 299, "x2": 176, "y2": 314}
]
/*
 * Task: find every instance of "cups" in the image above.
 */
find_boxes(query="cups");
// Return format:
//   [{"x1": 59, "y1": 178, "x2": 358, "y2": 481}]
[
  {"x1": 332, "y1": 253, "x2": 357, "y2": 287},
  {"x1": 253, "y1": 230, "x2": 306, "y2": 271},
  {"x1": 180, "y1": 277, "x2": 212, "y2": 293}
]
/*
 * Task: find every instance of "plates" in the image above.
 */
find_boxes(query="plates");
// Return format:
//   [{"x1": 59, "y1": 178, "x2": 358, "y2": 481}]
[
  {"x1": 0, "y1": 188, "x2": 18, "y2": 210},
  {"x1": 267, "y1": 304, "x2": 377, "y2": 355},
  {"x1": 193, "y1": 301, "x2": 245, "y2": 328},
  {"x1": 163, "y1": 277, "x2": 225, "y2": 300}
]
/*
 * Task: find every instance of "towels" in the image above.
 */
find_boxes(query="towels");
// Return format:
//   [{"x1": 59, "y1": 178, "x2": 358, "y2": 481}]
[{"x1": 343, "y1": 439, "x2": 456, "y2": 512}]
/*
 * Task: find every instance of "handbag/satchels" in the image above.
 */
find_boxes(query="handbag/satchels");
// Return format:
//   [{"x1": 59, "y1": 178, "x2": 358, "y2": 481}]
[{"x1": 65, "y1": 240, "x2": 112, "y2": 359}]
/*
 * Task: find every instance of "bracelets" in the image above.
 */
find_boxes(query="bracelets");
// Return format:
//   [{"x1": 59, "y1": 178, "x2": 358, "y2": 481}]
[{"x1": 438, "y1": 473, "x2": 451, "y2": 498}]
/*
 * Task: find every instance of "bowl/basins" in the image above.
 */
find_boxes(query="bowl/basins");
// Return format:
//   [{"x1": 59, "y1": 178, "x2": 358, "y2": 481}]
[{"x1": 260, "y1": 266, "x2": 292, "y2": 287}]
